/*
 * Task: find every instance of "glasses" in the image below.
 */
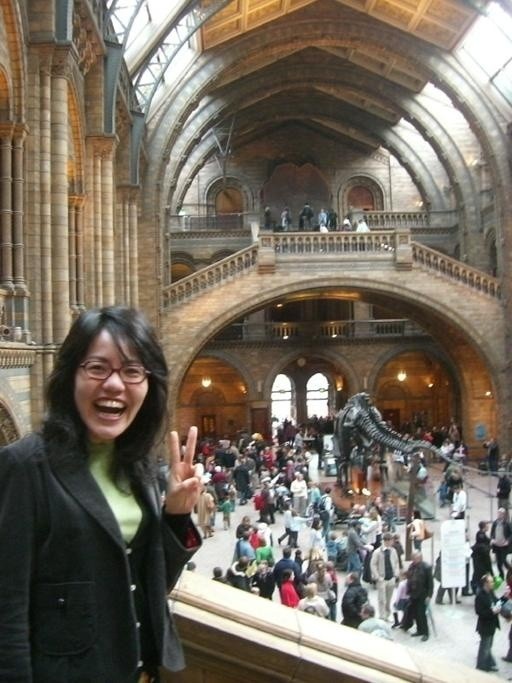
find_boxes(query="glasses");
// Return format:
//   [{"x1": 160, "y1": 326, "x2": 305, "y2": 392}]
[{"x1": 76, "y1": 359, "x2": 156, "y2": 385}]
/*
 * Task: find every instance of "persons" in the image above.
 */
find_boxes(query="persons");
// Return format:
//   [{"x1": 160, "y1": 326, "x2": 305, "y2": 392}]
[
  {"x1": 157, "y1": 409, "x2": 512, "y2": 673},
  {"x1": 0, "y1": 306, "x2": 206, "y2": 683},
  {"x1": 265, "y1": 202, "x2": 370, "y2": 250}
]
